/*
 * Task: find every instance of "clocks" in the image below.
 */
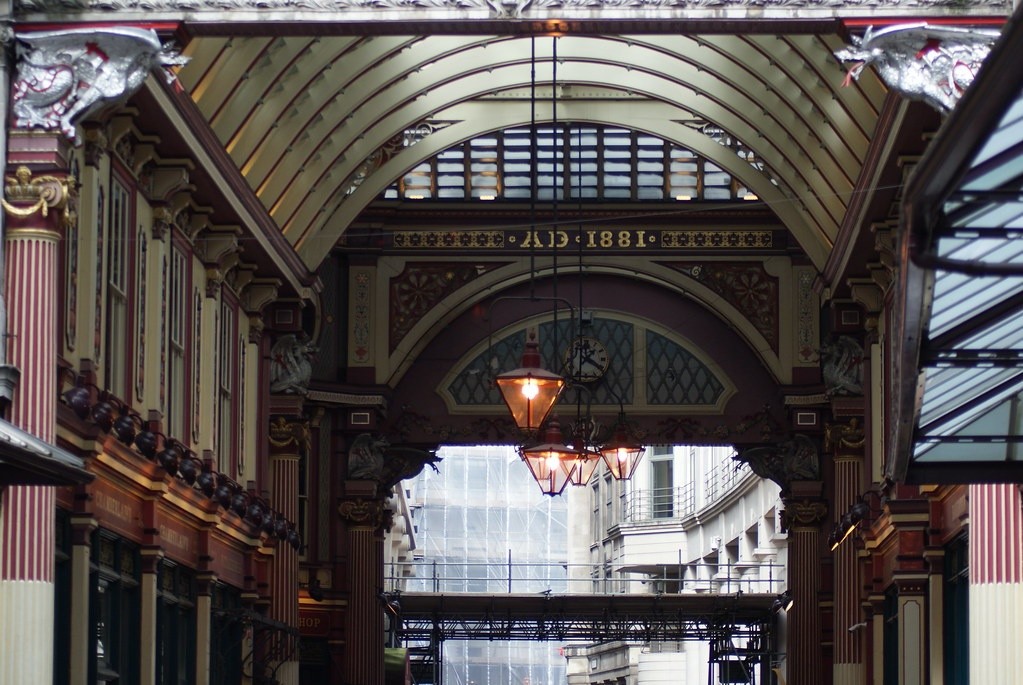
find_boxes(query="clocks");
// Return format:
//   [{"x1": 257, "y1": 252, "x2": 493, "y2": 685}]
[{"x1": 564, "y1": 337, "x2": 609, "y2": 383}]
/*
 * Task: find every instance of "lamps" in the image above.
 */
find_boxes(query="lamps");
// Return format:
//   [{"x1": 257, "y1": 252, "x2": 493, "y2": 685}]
[
  {"x1": 58, "y1": 364, "x2": 301, "y2": 551},
  {"x1": 827, "y1": 490, "x2": 883, "y2": 551},
  {"x1": 300, "y1": 578, "x2": 324, "y2": 602},
  {"x1": 495, "y1": 16, "x2": 647, "y2": 499}
]
